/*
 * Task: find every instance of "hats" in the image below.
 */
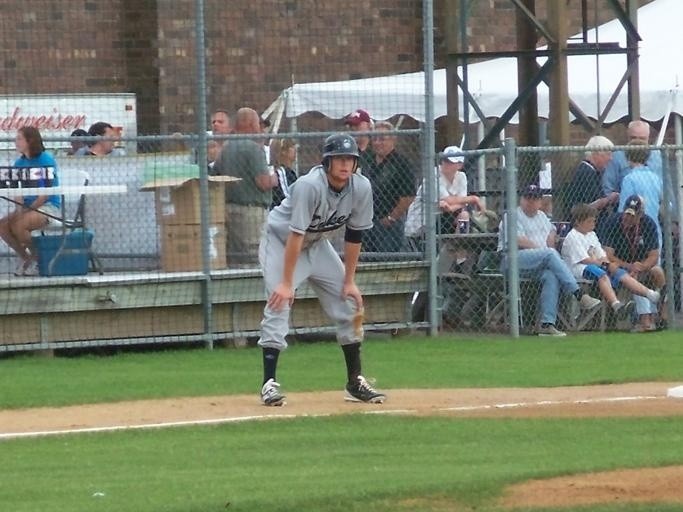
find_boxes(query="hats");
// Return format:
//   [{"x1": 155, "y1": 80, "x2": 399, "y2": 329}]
[
  {"x1": 623, "y1": 194, "x2": 645, "y2": 216},
  {"x1": 344, "y1": 109, "x2": 370, "y2": 126},
  {"x1": 444, "y1": 144, "x2": 466, "y2": 164},
  {"x1": 523, "y1": 183, "x2": 543, "y2": 198}
]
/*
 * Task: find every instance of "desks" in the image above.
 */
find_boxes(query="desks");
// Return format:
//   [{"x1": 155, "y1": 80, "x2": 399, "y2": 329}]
[{"x1": 1, "y1": 186, "x2": 125, "y2": 276}]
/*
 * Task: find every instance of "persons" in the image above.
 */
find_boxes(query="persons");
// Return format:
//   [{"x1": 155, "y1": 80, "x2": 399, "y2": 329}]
[
  {"x1": 255, "y1": 131, "x2": 388, "y2": 409},
  {"x1": 0, "y1": 125, "x2": 64, "y2": 279},
  {"x1": 405, "y1": 145, "x2": 485, "y2": 256},
  {"x1": 497, "y1": 186, "x2": 600, "y2": 336},
  {"x1": 562, "y1": 121, "x2": 668, "y2": 328},
  {"x1": 599, "y1": 194, "x2": 665, "y2": 330},
  {"x1": 72, "y1": 122, "x2": 125, "y2": 156},
  {"x1": 206, "y1": 106, "x2": 297, "y2": 265},
  {"x1": 561, "y1": 202, "x2": 668, "y2": 320},
  {"x1": 344, "y1": 109, "x2": 416, "y2": 252}
]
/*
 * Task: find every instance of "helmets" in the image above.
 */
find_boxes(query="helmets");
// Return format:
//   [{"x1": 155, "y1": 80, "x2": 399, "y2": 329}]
[{"x1": 322, "y1": 133, "x2": 360, "y2": 172}]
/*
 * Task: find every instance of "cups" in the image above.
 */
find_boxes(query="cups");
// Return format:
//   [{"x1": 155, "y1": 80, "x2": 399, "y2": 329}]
[
  {"x1": 458, "y1": 220, "x2": 469, "y2": 234},
  {"x1": 551, "y1": 222, "x2": 571, "y2": 237}
]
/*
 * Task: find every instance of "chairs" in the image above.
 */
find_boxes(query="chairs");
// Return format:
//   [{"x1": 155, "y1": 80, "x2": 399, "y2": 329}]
[{"x1": 40, "y1": 170, "x2": 105, "y2": 274}]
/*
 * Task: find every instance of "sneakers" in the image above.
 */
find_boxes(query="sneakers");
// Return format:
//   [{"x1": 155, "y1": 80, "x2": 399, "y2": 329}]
[
  {"x1": 260, "y1": 378, "x2": 288, "y2": 406},
  {"x1": 343, "y1": 376, "x2": 386, "y2": 403},
  {"x1": 579, "y1": 294, "x2": 601, "y2": 310},
  {"x1": 630, "y1": 322, "x2": 657, "y2": 332},
  {"x1": 24, "y1": 259, "x2": 40, "y2": 276},
  {"x1": 535, "y1": 325, "x2": 567, "y2": 336},
  {"x1": 614, "y1": 300, "x2": 635, "y2": 322},
  {"x1": 647, "y1": 289, "x2": 660, "y2": 303},
  {"x1": 13, "y1": 261, "x2": 26, "y2": 275}
]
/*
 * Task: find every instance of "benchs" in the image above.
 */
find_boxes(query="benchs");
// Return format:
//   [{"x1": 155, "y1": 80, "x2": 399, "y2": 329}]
[{"x1": 408, "y1": 215, "x2": 619, "y2": 333}]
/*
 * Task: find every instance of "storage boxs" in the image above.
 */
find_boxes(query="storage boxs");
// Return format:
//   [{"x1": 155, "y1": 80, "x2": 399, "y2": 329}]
[
  {"x1": 140, "y1": 172, "x2": 243, "y2": 224},
  {"x1": 158, "y1": 220, "x2": 227, "y2": 272},
  {"x1": 26, "y1": 232, "x2": 95, "y2": 274}
]
